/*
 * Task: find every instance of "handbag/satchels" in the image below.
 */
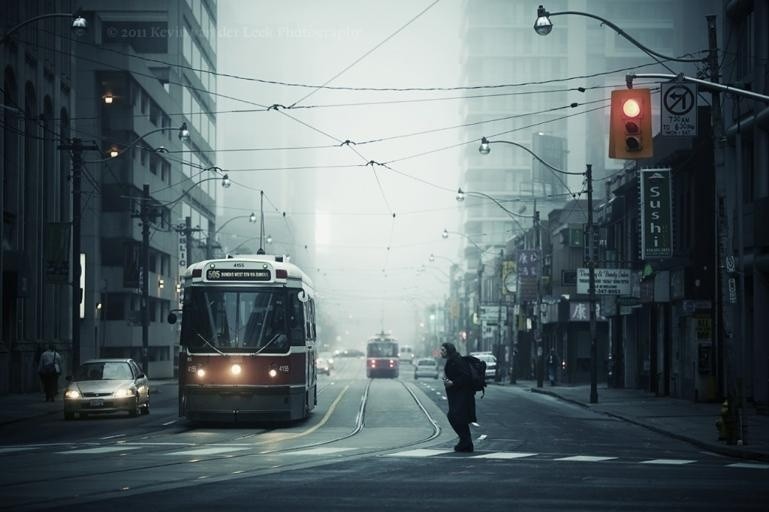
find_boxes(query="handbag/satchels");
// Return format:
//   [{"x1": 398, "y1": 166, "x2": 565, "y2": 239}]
[
  {"x1": 39, "y1": 364, "x2": 56, "y2": 379},
  {"x1": 462, "y1": 355, "x2": 487, "y2": 393}
]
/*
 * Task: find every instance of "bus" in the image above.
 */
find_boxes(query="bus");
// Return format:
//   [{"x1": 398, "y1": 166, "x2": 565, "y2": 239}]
[
  {"x1": 171, "y1": 254, "x2": 322, "y2": 431},
  {"x1": 364, "y1": 329, "x2": 399, "y2": 380}
]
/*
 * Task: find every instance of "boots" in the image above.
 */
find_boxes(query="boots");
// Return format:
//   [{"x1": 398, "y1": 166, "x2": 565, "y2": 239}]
[{"x1": 455, "y1": 439, "x2": 473, "y2": 452}]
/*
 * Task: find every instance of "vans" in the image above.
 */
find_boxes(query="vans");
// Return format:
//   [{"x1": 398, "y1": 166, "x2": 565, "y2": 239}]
[{"x1": 397, "y1": 343, "x2": 414, "y2": 364}]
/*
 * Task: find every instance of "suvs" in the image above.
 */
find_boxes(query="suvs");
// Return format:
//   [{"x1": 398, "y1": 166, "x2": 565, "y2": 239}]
[{"x1": 413, "y1": 358, "x2": 439, "y2": 380}]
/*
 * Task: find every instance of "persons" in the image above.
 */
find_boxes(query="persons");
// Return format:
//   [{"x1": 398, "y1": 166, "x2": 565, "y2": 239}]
[
  {"x1": 38, "y1": 342, "x2": 62, "y2": 402},
  {"x1": 441, "y1": 342, "x2": 477, "y2": 453}
]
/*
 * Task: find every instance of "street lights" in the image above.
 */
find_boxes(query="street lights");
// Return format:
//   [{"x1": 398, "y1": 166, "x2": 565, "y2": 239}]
[
  {"x1": 222, "y1": 234, "x2": 274, "y2": 255},
  {"x1": 532, "y1": 4, "x2": 744, "y2": 446},
  {"x1": 129, "y1": 176, "x2": 233, "y2": 373},
  {"x1": 417, "y1": 226, "x2": 484, "y2": 289},
  {"x1": 70, "y1": 120, "x2": 197, "y2": 367},
  {"x1": 211, "y1": 211, "x2": 258, "y2": 237},
  {"x1": 451, "y1": 187, "x2": 546, "y2": 390},
  {"x1": 1, "y1": 11, "x2": 88, "y2": 44},
  {"x1": 474, "y1": 133, "x2": 601, "y2": 405}
]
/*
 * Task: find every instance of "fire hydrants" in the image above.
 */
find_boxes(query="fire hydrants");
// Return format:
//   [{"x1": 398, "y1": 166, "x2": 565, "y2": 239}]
[{"x1": 715, "y1": 400, "x2": 729, "y2": 443}]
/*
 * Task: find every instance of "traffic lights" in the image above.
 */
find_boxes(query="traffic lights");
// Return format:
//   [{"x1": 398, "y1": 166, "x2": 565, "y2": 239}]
[{"x1": 605, "y1": 87, "x2": 654, "y2": 162}]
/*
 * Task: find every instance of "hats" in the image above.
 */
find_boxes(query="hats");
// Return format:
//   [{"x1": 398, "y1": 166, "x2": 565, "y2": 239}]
[{"x1": 441, "y1": 343, "x2": 456, "y2": 359}]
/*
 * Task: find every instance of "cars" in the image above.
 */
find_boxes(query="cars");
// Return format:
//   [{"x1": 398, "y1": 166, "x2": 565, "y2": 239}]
[
  {"x1": 471, "y1": 354, "x2": 496, "y2": 379},
  {"x1": 315, "y1": 348, "x2": 363, "y2": 376},
  {"x1": 59, "y1": 355, "x2": 151, "y2": 421}
]
